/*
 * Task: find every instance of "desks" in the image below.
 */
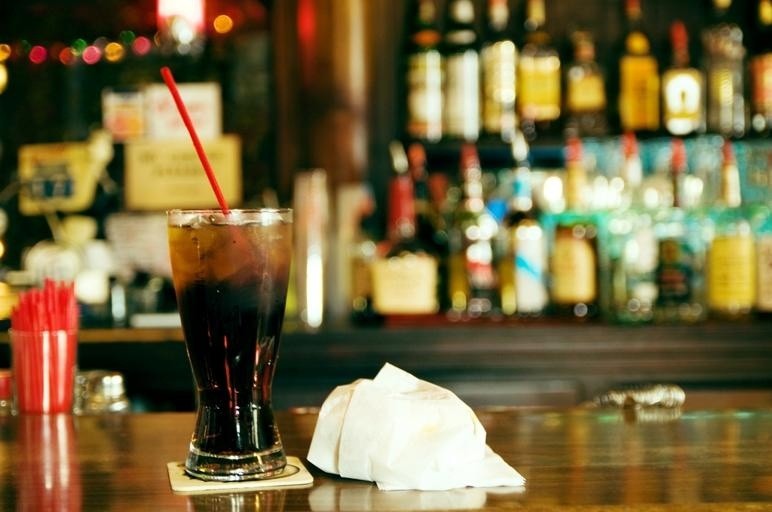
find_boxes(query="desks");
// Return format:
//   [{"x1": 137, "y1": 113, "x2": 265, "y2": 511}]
[{"x1": 3, "y1": 382, "x2": 772, "y2": 511}]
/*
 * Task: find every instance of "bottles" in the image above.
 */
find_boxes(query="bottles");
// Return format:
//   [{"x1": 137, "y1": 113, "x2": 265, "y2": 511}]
[{"x1": 369, "y1": 1, "x2": 772, "y2": 326}]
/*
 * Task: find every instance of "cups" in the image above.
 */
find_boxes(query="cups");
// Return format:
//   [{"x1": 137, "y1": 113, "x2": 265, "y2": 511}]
[
  {"x1": 7, "y1": 331, "x2": 78, "y2": 415},
  {"x1": 166, "y1": 207, "x2": 293, "y2": 480}
]
